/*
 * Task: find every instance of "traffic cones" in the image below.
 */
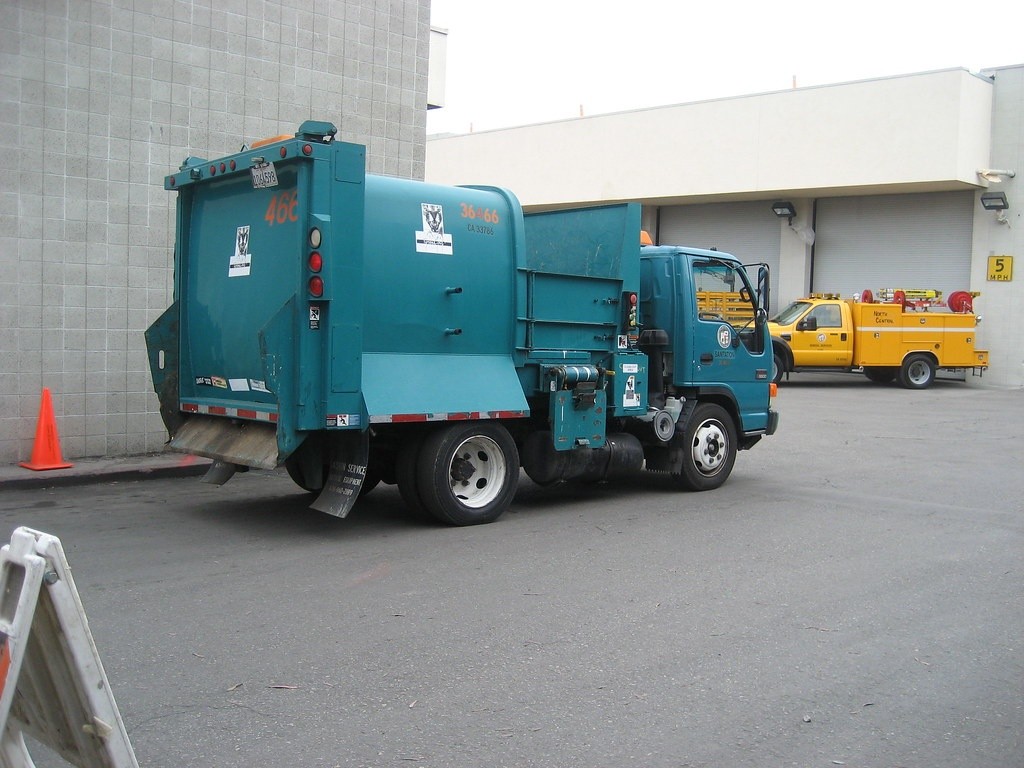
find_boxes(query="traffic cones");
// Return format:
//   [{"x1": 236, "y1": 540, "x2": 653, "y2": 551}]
[{"x1": 19, "y1": 386, "x2": 75, "y2": 471}]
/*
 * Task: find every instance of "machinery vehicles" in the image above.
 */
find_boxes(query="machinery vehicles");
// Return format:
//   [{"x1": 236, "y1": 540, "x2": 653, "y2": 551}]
[
  {"x1": 722, "y1": 287, "x2": 990, "y2": 390},
  {"x1": 144, "y1": 119, "x2": 781, "y2": 528}
]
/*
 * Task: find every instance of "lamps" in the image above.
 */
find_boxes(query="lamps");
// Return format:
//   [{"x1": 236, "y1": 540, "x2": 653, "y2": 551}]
[
  {"x1": 773, "y1": 201, "x2": 796, "y2": 218},
  {"x1": 981, "y1": 191, "x2": 1009, "y2": 210}
]
